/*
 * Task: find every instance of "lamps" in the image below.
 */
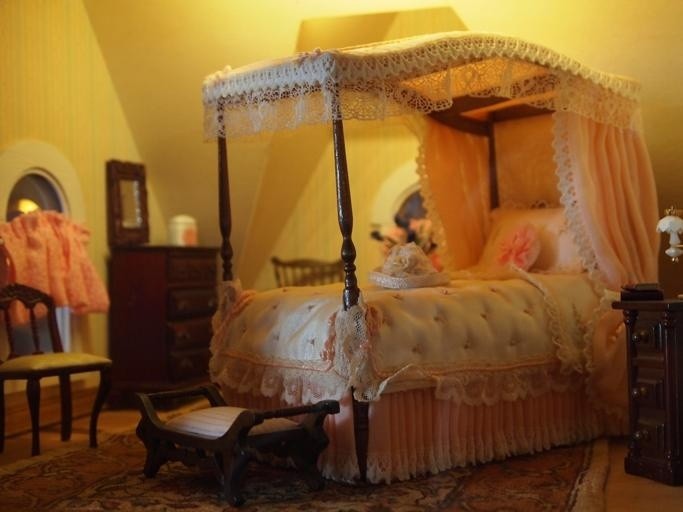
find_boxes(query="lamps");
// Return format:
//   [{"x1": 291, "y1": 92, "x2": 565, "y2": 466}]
[{"x1": 656, "y1": 205, "x2": 683, "y2": 264}]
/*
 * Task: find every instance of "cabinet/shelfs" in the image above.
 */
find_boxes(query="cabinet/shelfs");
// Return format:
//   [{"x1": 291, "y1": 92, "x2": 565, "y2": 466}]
[{"x1": 89, "y1": 246, "x2": 218, "y2": 449}]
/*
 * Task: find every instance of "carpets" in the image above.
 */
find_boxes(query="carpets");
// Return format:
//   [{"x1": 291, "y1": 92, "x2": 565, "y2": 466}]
[{"x1": 0, "y1": 389, "x2": 609, "y2": 512}]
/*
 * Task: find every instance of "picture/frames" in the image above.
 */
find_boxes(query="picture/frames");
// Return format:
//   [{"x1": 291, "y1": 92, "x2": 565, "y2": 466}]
[{"x1": 106, "y1": 160, "x2": 149, "y2": 246}]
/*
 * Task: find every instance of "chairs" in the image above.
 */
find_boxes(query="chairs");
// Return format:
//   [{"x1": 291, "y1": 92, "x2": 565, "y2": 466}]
[{"x1": 0, "y1": 282, "x2": 115, "y2": 457}]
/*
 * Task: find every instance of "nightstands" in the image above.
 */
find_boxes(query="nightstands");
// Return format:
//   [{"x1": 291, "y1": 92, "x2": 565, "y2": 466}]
[{"x1": 612, "y1": 283, "x2": 683, "y2": 487}]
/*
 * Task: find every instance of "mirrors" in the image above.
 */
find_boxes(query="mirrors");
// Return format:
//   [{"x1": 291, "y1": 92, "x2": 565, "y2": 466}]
[{"x1": 0, "y1": 142, "x2": 89, "y2": 381}]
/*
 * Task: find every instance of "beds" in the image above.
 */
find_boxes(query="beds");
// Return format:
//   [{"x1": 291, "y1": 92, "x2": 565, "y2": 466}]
[{"x1": 201, "y1": 31, "x2": 669, "y2": 488}]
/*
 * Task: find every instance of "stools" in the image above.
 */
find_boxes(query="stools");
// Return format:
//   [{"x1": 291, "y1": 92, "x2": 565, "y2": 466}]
[{"x1": 133, "y1": 381, "x2": 340, "y2": 507}]
